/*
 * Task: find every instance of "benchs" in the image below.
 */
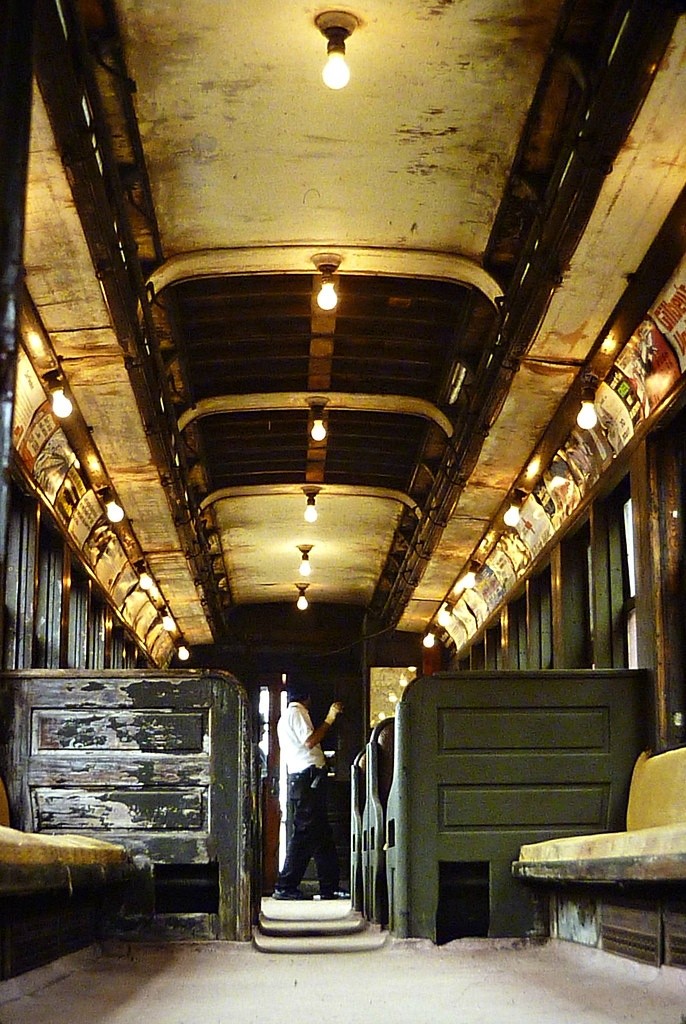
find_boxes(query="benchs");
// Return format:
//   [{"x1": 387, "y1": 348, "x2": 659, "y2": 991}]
[
  {"x1": 508, "y1": 748, "x2": 686, "y2": 970},
  {"x1": 0, "y1": 778, "x2": 130, "y2": 1003},
  {"x1": 0, "y1": 666, "x2": 655, "y2": 944}
]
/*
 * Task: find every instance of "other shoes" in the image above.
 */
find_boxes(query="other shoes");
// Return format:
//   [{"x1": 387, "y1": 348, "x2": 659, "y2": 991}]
[
  {"x1": 321, "y1": 888, "x2": 351, "y2": 900},
  {"x1": 271, "y1": 886, "x2": 314, "y2": 900}
]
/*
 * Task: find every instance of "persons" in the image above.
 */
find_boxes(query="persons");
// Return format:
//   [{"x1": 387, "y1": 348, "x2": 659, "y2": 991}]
[
  {"x1": 257, "y1": 713, "x2": 266, "y2": 763},
  {"x1": 272, "y1": 685, "x2": 350, "y2": 900}
]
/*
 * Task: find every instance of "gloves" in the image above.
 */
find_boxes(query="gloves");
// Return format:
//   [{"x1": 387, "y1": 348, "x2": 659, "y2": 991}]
[{"x1": 324, "y1": 702, "x2": 345, "y2": 726}]
